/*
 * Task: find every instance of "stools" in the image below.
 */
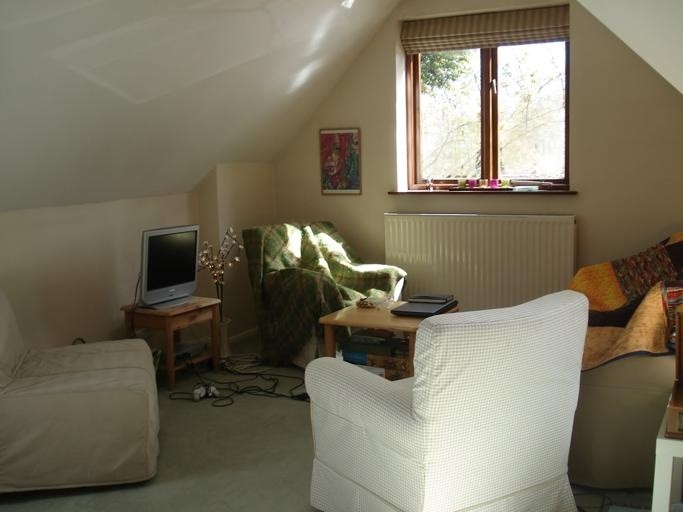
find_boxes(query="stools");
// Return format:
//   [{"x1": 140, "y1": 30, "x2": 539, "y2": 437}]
[{"x1": 121, "y1": 295, "x2": 222, "y2": 390}]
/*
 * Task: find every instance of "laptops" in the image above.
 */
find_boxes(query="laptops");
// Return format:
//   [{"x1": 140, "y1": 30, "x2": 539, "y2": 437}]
[{"x1": 391, "y1": 299, "x2": 458, "y2": 317}]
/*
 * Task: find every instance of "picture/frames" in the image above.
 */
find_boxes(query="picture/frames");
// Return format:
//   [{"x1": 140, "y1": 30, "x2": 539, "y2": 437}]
[{"x1": 318, "y1": 127, "x2": 362, "y2": 194}]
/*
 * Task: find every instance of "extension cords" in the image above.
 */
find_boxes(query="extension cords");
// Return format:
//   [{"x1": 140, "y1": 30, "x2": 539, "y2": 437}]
[{"x1": 194, "y1": 385, "x2": 217, "y2": 401}]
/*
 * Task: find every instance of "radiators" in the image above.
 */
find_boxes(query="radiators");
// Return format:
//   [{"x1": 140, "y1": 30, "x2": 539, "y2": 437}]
[{"x1": 384, "y1": 212, "x2": 580, "y2": 311}]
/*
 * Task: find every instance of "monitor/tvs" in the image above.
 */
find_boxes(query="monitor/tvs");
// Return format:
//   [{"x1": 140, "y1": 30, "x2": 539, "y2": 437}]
[{"x1": 141, "y1": 222, "x2": 201, "y2": 309}]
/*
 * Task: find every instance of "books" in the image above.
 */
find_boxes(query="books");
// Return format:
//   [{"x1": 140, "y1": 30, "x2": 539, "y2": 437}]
[{"x1": 407, "y1": 290, "x2": 455, "y2": 305}]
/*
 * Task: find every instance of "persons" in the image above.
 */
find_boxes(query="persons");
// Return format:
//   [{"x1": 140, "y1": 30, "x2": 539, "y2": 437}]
[{"x1": 320, "y1": 133, "x2": 353, "y2": 188}]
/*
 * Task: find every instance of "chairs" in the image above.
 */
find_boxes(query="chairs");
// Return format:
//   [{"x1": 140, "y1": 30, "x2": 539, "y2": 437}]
[
  {"x1": 0, "y1": 282, "x2": 161, "y2": 492},
  {"x1": 242, "y1": 221, "x2": 407, "y2": 367},
  {"x1": 566, "y1": 230, "x2": 683, "y2": 490},
  {"x1": 305, "y1": 290, "x2": 589, "y2": 512}
]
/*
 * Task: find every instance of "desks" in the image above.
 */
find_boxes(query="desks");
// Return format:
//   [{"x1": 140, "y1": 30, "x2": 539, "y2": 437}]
[
  {"x1": 318, "y1": 300, "x2": 459, "y2": 377},
  {"x1": 651, "y1": 392, "x2": 683, "y2": 512}
]
